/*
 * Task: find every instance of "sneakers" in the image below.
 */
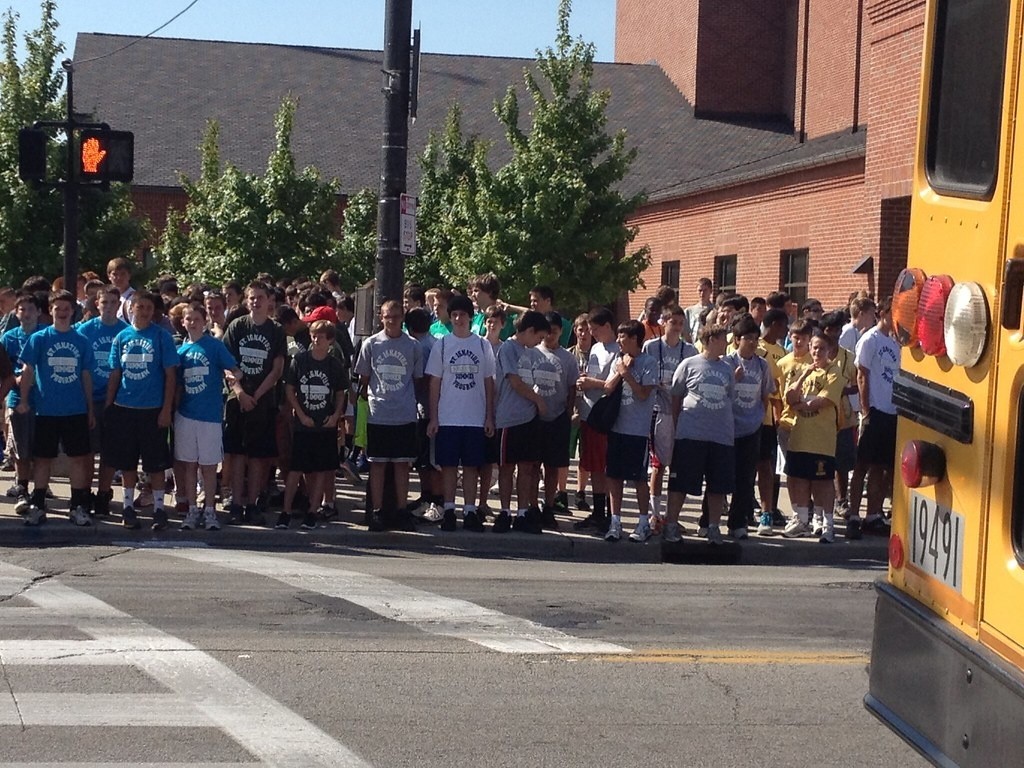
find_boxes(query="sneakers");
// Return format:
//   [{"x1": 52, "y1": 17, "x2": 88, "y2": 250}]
[{"x1": 1, "y1": 458, "x2": 893, "y2": 548}]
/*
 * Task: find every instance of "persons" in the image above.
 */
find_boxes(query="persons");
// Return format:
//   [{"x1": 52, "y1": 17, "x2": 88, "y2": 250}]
[{"x1": 1, "y1": 256, "x2": 898, "y2": 542}]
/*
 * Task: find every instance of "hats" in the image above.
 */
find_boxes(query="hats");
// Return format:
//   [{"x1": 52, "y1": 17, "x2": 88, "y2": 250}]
[{"x1": 301, "y1": 306, "x2": 336, "y2": 324}]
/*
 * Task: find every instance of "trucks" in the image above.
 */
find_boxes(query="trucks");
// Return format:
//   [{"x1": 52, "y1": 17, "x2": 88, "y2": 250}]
[{"x1": 863, "y1": 1, "x2": 1024, "y2": 763}]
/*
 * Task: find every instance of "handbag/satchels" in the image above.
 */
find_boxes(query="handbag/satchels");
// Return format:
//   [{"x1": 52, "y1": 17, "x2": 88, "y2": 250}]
[{"x1": 585, "y1": 378, "x2": 623, "y2": 435}]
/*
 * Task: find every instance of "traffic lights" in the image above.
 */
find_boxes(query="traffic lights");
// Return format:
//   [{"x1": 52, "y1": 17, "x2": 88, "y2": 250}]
[
  {"x1": 76, "y1": 117, "x2": 135, "y2": 191},
  {"x1": 15, "y1": 121, "x2": 68, "y2": 196}
]
